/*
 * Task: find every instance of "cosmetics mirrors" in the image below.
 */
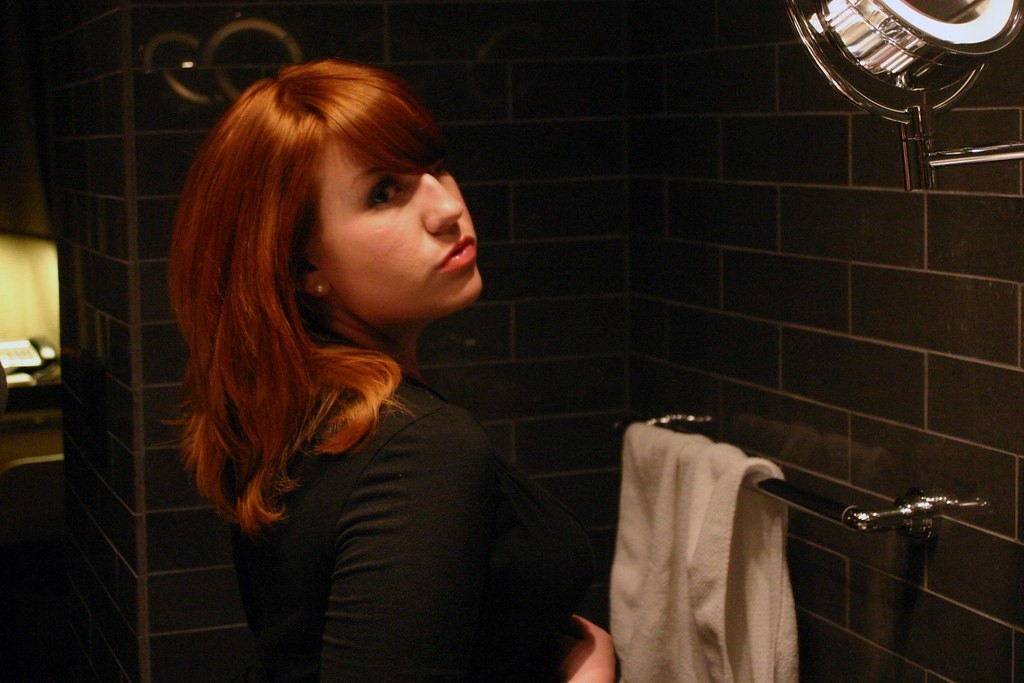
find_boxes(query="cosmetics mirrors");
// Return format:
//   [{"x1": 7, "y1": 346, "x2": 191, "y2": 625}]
[{"x1": 787, "y1": 1, "x2": 1023, "y2": 193}]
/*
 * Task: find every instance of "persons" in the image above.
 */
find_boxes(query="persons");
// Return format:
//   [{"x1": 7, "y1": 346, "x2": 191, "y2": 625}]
[{"x1": 165, "y1": 54, "x2": 617, "y2": 683}]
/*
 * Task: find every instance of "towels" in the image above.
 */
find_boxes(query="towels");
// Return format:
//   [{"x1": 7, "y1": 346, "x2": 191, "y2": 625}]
[{"x1": 609, "y1": 423, "x2": 799, "y2": 683}]
